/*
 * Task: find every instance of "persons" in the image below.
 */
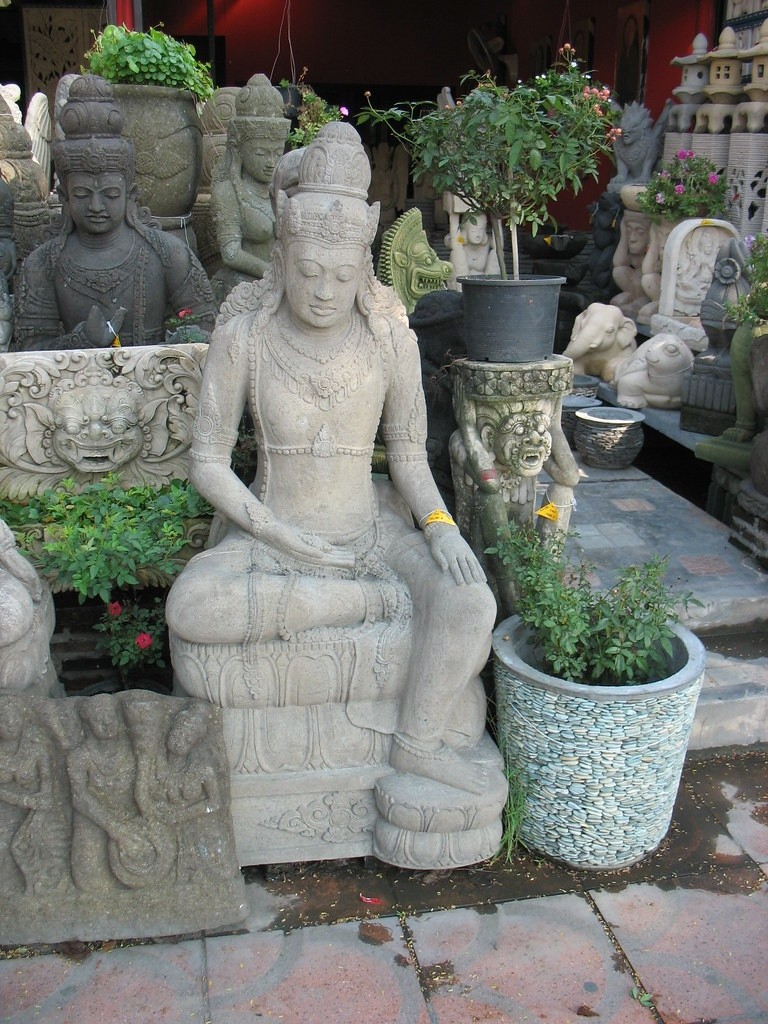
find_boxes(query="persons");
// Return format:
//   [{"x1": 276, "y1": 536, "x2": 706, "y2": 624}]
[
  {"x1": 610, "y1": 210, "x2": 661, "y2": 325},
  {"x1": 677, "y1": 228, "x2": 728, "y2": 317},
  {"x1": 443, "y1": 210, "x2": 499, "y2": 292},
  {"x1": 15, "y1": 73, "x2": 219, "y2": 351},
  {"x1": 165, "y1": 121, "x2": 498, "y2": 796},
  {"x1": 448, "y1": 375, "x2": 581, "y2": 610},
  {"x1": 212, "y1": 73, "x2": 290, "y2": 292}
]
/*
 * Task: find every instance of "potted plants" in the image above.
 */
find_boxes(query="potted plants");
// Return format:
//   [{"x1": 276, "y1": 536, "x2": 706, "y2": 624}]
[
  {"x1": 483, "y1": 519, "x2": 706, "y2": 871},
  {"x1": 80, "y1": 19, "x2": 219, "y2": 217}
]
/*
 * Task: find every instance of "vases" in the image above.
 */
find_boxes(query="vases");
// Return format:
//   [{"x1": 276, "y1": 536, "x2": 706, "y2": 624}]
[
  {"x1": 79, "y1": 680, "x2": 172, "y2": 698},
  {"x1": 573, "y1": 406, "x2": 647, "y2": 470},
  {"x1": 274, "y1": 84, "x2": 303, "y2": 118},
  {"x1": 568, "y1": 372, "x2": 600, "y2": 399},
  {"x1": 456, "y1": 273, "x2": 567, "y2": 363},
  {"x1": 560, "y1": 396, "x2": 602, "y2": 449},
  {"x1": 676, "y1": 202, "x2": 708, "y2": 217}
]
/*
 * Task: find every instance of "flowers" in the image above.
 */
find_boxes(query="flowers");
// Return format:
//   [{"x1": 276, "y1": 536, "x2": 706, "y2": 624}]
[
  {"x1": 635, "y1": 151, "x2": 734, "y2": 223},
  {"x1": 94, "y1": 599, "x2": 167, "y2": 690},
  {"x1": 279, "y1": 79, "x2": 349, "y2": 148},
  {"x1": 356, "y1": 42, "x2": 622, "y2": 279}
]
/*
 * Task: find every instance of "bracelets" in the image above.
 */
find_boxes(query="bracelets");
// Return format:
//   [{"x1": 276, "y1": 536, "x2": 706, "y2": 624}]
[{"x1": 419, "y1": 509, "x2": 453, "y2": 528}]
